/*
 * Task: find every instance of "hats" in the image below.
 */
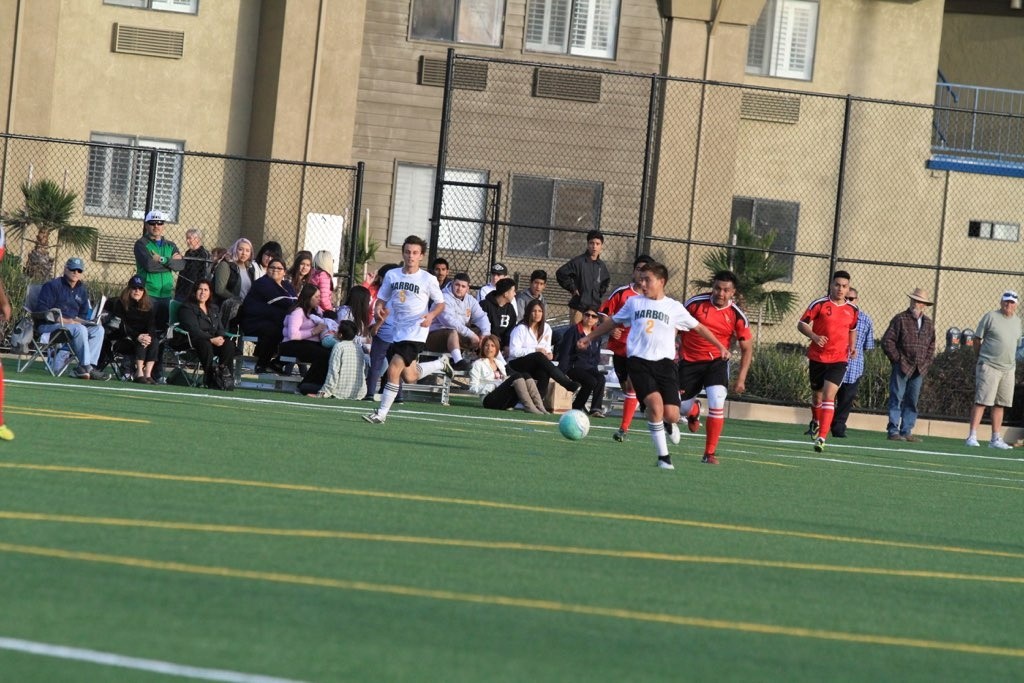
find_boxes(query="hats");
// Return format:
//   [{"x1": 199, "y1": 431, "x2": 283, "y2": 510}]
[
  {"x1": 908, "y1": 288, "x2": 935, "y2": 306},
  {"x1": 67, "y1": 257, "x2": 84, "y2": 271},
  {"x1": 145, "y1": 210, "x2": 165, "y2": 220},
  {"x1": 491, "y1": 263, "x2": 507, "y2": 275},
  {"x1": 1002, "y1": 291, "x2": 1018, "y2": 303},
  {"x1": 129, "y1": 275, "x2": 144, "y2": 287}
]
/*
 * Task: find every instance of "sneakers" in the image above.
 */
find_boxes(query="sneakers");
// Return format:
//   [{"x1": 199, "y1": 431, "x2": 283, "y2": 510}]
[
  {"x1": 988, "y1": 438, "x2": 1013, "y2": 450},
  {"x1": 663, "y1": 420, "x2": 680, "y2": 445},
  {"x1": 657, "y1": 456, "x2": 673, "y2": 469},
  {"x1": 702, "y1": 453, "x2": 719, "y2": 464},
  {"x1": 613, "y1": 429, "x2": 626, "y2": 441},
  {"x1": 686, "y1": 400, "x2": 702, "y2": 433},
  {"x1": 966, "y1": 435, "x2": 980, "y2": 446}
]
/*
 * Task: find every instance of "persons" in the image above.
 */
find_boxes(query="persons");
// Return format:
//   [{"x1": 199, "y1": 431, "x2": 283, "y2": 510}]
[
  {"x1": 0, "y1": 209, "x2": 873, "y2": 469},
  {"x1": 967, "y1": 291, "x2": 1023, "y2": 450},
  {"x1": 880, "y1": 288, "x2": 941, "y2": 443}
]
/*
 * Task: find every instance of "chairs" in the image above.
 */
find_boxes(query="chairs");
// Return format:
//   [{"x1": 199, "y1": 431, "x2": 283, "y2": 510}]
[
  {"x1": 18, "y1": 285, "x2": 169, "y2": 382},
  {"x1": 167, "y1": 300, "x2": 240, "y2": 387}
]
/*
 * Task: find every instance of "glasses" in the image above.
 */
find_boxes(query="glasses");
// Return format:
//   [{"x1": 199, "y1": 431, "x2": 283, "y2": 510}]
[
  {"x1": 1003, "y1": 293, "x2": 1018, "y2": 299},
  {"x1": 846, "y1": 296, "x2": 857, "y2": 301},
  {"x1": 145, "y1": 220, "x2": 164, "y2": 225},
  {"x1": 270, "y1": 266, "x2": 283, "y2": 271},
  {"x1": 584, "y1": 313, "x2": 598, "y2": 319}
]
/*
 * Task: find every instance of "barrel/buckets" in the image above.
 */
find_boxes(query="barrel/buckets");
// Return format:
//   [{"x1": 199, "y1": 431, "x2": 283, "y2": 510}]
[{"x1": 45, "y1": 343, "x2": 70, "y2": 375}]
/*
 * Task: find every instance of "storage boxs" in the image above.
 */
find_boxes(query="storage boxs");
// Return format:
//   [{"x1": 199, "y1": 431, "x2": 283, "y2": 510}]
[{"x1": 547, "y1": 382, "x2": 573, "y2": 413}]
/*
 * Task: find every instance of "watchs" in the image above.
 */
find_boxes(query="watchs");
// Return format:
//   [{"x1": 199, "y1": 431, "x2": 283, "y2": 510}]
[{"x1": 159, "y1": 257, "x2": 165, "y2": 263}]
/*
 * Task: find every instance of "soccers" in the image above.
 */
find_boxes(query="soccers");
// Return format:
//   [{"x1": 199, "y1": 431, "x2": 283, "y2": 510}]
[{"x1": 558, "y1": 409, "x2": 590, "y2": 440}]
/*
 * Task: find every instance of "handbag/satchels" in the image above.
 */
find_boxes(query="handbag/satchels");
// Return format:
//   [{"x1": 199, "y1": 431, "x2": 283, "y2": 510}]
[
  {"x1": 45, "y1": 348, "x2": 67, "y2": 377},
  {"x1": 204, "y1": 356, "x2": 234, "y2": 390}
]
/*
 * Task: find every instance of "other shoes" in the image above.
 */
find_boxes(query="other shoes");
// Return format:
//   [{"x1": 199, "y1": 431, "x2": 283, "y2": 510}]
[
  {"x1": 888, "y1": 432, "x2": 924, "y2": 443},
  {"x1": 804, "y1": 421, "x2": 849, "y2": 453},
  {"x1": 589, "y1": 411, "x2": 604, "y2": 418},
  {"x1": 0, "y1": 424, "x2": 15, "y2": 439},
  {"x1": 362, "y1": 354, "x2": 472, "y2": 425},
  {"x1": 69, "y1": 357, "x2": 287, "y2": 386}
]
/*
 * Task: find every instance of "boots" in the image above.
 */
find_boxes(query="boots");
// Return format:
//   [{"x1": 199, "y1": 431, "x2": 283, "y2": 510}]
[
  {"x1": 550, "y1": 367, "x2": 581, "y2": 393},
  {"x1": 524, "y1": 378, "x2": 550, "y2": 414},
  {"x1": 510, "y1": 378, "x2": 543, "y2": 415}
]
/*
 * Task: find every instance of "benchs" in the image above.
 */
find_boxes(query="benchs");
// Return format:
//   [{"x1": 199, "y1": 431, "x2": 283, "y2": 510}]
[{"x1": 236, "y1": 336, "x2": 622, "y2": 413}]
